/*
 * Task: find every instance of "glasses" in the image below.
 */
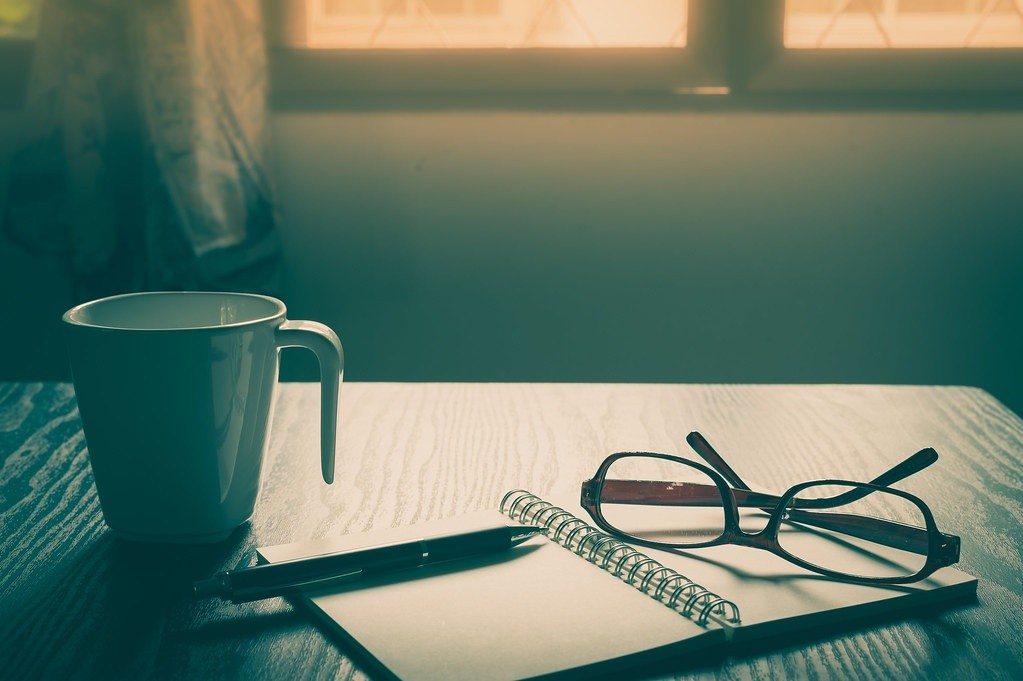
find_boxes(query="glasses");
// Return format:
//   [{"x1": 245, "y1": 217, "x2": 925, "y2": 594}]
[{"x1": 581, "y1": 431, "x2": 961, "y2": 585}]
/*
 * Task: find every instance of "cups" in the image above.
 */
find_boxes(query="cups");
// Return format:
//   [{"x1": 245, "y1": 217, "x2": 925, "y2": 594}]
[{"x1": 62, "y1": 291, "x2": 346, "y2": 546}]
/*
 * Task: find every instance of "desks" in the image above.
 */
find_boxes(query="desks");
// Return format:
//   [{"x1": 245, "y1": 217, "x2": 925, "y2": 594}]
[{"x1": 0, "y1": 379, "x2": 1023, "y2": 681}]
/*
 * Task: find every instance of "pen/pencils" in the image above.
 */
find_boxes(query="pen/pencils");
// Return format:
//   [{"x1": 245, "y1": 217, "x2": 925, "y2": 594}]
[{"x1": 191, "y1": 525, "x2": 551, "y2": 606}]
